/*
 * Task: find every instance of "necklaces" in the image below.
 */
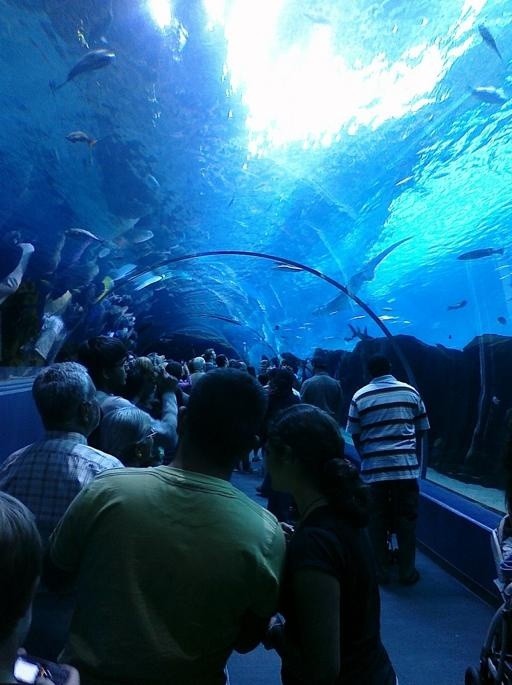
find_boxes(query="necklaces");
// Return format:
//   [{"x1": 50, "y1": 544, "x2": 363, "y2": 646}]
[{"x1": 302, "y1": 497, "x2": 327, "y2": 514}]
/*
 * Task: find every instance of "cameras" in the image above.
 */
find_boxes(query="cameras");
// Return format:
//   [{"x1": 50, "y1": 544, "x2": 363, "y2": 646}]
[{"x1": 12, "y1": 652, "x2": 70, "y2": 685}]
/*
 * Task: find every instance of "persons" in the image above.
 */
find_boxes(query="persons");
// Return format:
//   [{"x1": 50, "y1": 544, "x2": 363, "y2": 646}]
[
  {"x1": 1, "y1": 335, "x2": 430, "y2": 685},
  {"x1": 497, "y1": 483, "x2": 511, "y2": 599}
]
